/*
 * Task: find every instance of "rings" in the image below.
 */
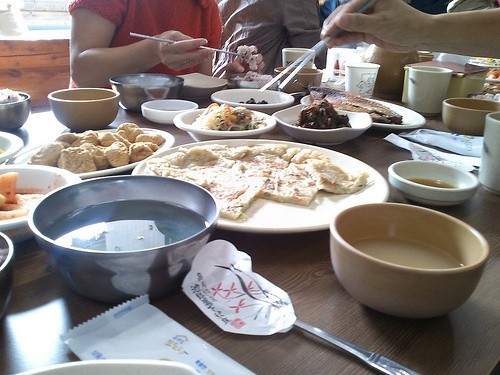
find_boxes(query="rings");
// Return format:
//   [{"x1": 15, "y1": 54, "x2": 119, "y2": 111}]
[{"x1": 184, "y1": 59, "x2": 186, "y2": 65}]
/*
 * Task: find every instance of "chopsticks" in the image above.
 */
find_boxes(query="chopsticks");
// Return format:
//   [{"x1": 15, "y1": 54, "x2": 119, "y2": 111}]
[
  {"x1": 258, "y1": 1, "x2": 378, "y2": 92},
  {"x1": 128, "y1": 31, "x2": 249, "y2": 58}
]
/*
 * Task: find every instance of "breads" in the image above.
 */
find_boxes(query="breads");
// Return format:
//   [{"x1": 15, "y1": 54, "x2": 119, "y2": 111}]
[{"x1": 28, "y1": 123, "x2": 165, "y2": 173}]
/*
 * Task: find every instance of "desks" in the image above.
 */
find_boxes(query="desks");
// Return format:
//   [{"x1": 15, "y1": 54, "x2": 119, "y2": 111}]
[{"x1": 0, "y1": 87, "x2": 500, "y2": 375}]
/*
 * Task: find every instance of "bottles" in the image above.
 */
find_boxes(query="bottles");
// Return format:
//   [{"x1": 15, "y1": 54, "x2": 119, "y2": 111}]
[{"x1": 332, "y1": 52, "x2": 340, "y2": 77}]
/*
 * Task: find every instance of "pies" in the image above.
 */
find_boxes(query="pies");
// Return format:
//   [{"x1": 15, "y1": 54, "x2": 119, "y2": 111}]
[{"x1": 145, "y1": 142, "x2": 367, "y2": 222}]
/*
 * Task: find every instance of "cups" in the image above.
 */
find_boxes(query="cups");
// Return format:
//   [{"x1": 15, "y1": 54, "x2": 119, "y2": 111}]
[
  {"x1": 479, "y1": 111, "x2": 500, "y2": 196},
  {"x1": 408, "y1": 66, "x2": 453, "y2": 115},
  {"x1": 282, "y1": 47, "x2": 315, "y2": 69},
  {"x1": 343, "y1": 62, "x2": 382, "y2": 97}
]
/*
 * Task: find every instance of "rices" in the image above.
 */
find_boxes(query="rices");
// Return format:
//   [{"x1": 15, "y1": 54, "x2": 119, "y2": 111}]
[{"x1": 234, "y1": 45, "x2": 274, "y2": 81}]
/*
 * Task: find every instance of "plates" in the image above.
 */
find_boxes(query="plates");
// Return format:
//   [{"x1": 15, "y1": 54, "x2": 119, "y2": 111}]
[
  {"x1": 271, "y1": 104, "x2": 373, "y2": 146},
  {"x1": 0, "y1": 131, "x2": 24, "y2": 162},
  {"x1": 301, "y1": 91, "x2": 427, "y2": 128},
  {"x1": 175, "y1": 72, "x2": 228, "y2": 99},
  {"x1": 212, "y1": 85, "x2": 295, "y2": 113},
  {"x1": 14, "y1": 127, "x2": 175, "y2": 177},
  {"x1": 0, "y1": 165, "x2": 83, "y2": 241},
  {"x1": 132, "y1": 139, "x2": 391, "y2": 233}
]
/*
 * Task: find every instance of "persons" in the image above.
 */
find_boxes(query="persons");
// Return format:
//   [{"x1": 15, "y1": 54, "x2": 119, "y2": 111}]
[
  {"x1": 212, "y1": 0, "x2": 322, "y2": 86},
  {"x1": 68, "y1": 0, "x2": 263, "y2": 98},
  {"x1": 317, "y1": 0, "x2": 500, "y2": 60}
]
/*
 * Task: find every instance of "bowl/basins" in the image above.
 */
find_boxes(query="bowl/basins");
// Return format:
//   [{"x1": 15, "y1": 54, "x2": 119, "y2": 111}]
[
  {"x1": 0, "y1": 230, "x2": 15, "y2": 307},
  {"x1": 387, "y1": 160, "x2": 479, "y2": 206},
  {"x1": 330, "y1": 202, "x2": 491, "y2": 321},
  {"x1": 0, "y1": 89, "x2": 31, "y2": 131},
  {"x1": 109, "y1": 73, "x2": 184, "y2": 112},
  {"x1": 47, "y1": 87, "x2": 120, "y2": 131},
  {"x1": 137, "y1": 97, "x2": 199, "y2": 123},
  {"x1": 442, "y1": 98, "x2": 500, "y2": 135},
  {"x1": 400, "y1": 65, "x2": 488, "y2": 102},
  {"x1": 10, "y1": 357, "x2": 199, "y2": 375},
  {"x1": 30, "y1": 174, "x2": 219, "y2": 304},
  {"x1": 225, "y1": 73, "x2": 277, "y2": 89},
  {"x1": 274, "y1": 66, "x2": 324, "y2": 93}
]
